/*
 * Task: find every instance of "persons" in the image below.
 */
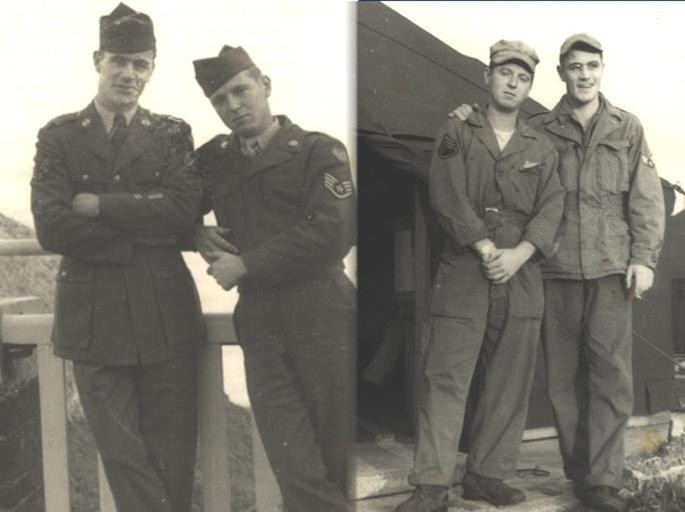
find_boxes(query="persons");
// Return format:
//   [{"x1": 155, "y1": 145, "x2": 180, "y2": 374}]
[
  {"x1": 29, "y1": 4, "x2": 204, "y2": 511},
  {"x1": 184, "y1": 46, "x2": 356, "y2": 510},
  {"x1": 446, "y1": 32, "x2": 668, "y2": 512},
  {"x1": 394, "y1": 39, "x2": 567, "y2": 512}
]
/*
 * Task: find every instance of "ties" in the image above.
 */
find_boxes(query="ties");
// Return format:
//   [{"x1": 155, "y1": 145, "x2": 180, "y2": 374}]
[{"x1": 108, "y1": 114, "x2": 132, "y2": 157}]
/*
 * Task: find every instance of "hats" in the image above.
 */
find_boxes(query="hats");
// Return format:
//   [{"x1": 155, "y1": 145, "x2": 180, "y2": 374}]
[
  {"x1": 558, "y1": 30, "x2": 603, "y2": 64},
  {"x1": 191, "y1": 44, "x2": 257, "y2": 100},
  {"x1": 97, "y1": 1, "x2": 157, "y2": 56},
  {"x1": 488, "y1": 37, "x2": 539, "y2": 74}
]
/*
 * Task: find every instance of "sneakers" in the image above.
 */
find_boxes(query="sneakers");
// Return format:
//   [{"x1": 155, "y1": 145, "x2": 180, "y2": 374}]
[
  {"x1": 460, "y1": 469, "x2": 526, "y2": 505},
  {"x1": 572, "y1": 476, "x2": 590, "y2": 502},
  {"x1": 394, "y1": 484, "x2": 451, "y2": 512},
  {"x1": 586, "y1": 485, "x2": 626, "y2": 512}
]
